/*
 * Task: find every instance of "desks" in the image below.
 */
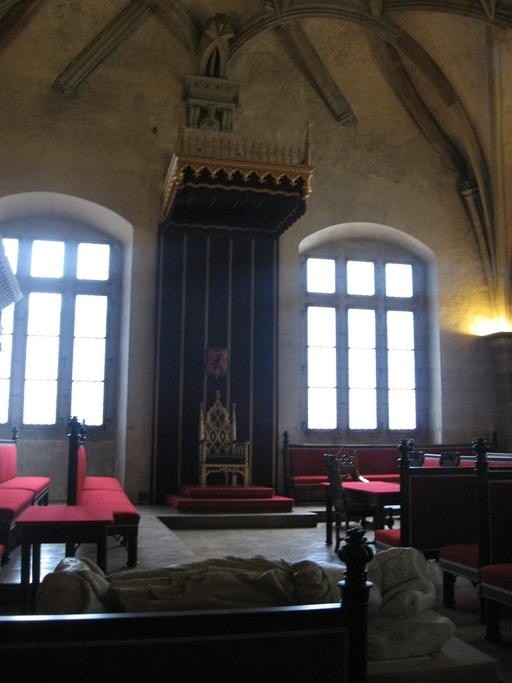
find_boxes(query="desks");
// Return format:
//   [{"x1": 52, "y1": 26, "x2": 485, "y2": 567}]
[{"x1": 319, "y1": 480, "x2": 404, "y2": 547}]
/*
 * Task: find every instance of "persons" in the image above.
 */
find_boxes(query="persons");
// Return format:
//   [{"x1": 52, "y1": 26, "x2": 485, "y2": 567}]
[{"x1": 197, "y1": 105, "x2": 221, "y2": 129}]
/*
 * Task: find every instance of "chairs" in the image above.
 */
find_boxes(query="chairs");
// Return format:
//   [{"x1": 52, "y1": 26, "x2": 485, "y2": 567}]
[
  {"x1": 198, "y1": 390, "x2": 253, "y2": 487},
  {"x1": 333, "y1": 447, "x2": 394, "y2": 532},
  {"x1": 1, "y1": 418, "x2": 141, "y2": 586},
  {"x1": 321, "y1": 452, "x2": 379, "y2": 551}
]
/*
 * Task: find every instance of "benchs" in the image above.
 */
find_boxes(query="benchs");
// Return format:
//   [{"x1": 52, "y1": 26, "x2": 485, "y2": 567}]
[
  {"x1": 437, "y1": 451, "x2": 510, "y2": 623},
  {"x1": 409, "y1": 438, "x2": 512, "y2": 468},
  {"x1": 0, "y1": 523, "x2": 375, "y2": 682},
  {"x1": 374, "y1": 441, "x2": 510, "y2": 554},
  {"x1": 284, "y1": 429, "x2": 498, "y2": 503},
  {"x1": 478, "y1": 560, "x2": 512, "y2": 642}
]
